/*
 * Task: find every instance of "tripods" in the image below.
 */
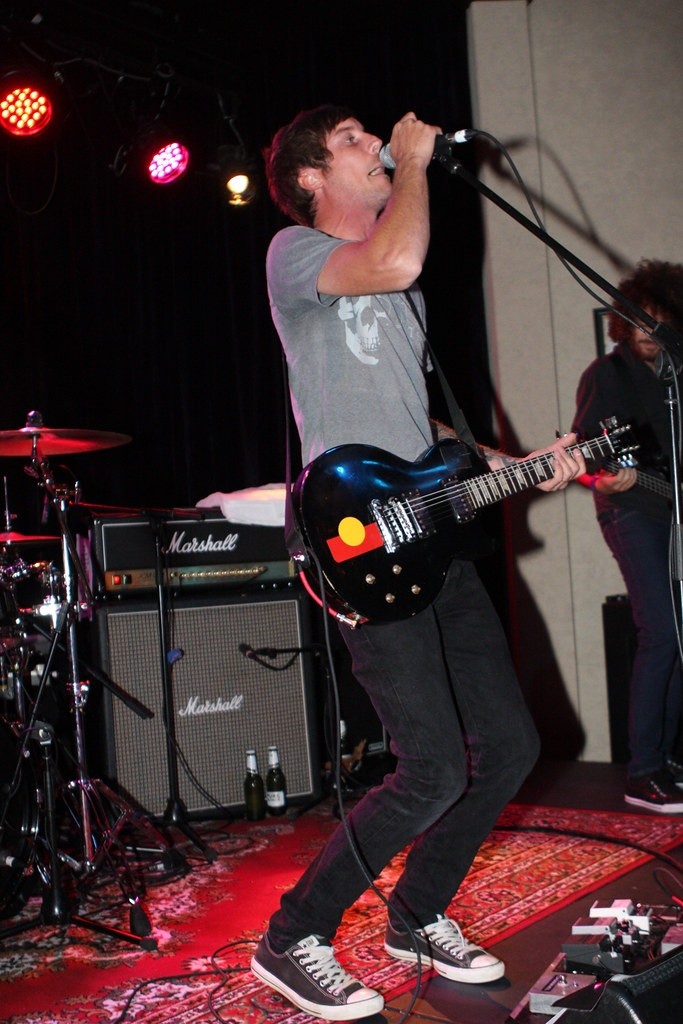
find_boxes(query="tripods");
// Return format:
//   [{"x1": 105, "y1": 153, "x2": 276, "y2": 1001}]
[
  {"x1": 253, "y1": 644, "x2": 364, "y2": 822},
  {"x1": 0, "y1": 440, "x2": 247, "y2": 950}
]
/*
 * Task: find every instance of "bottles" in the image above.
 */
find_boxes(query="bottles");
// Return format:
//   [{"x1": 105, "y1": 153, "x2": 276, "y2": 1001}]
[
  {"x1": 243, "y1": 750, "x2": 265, "y2": 822},
  {"x1": 266, "y1": 747, "x2": 287, "y2": 817}
]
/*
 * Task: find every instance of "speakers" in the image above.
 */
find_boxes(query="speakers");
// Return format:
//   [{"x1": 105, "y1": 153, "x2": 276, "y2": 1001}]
[
  {"x1": 75, "y1": 589, "x2": 324, "y2": 828},
  {"x1": 582, "y1": 944, "x2": 683, "y2": 1024}
]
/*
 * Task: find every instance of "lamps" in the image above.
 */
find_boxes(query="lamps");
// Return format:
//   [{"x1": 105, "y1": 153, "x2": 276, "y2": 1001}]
[
  {"x1": 0, "y1": 67, "x2": 59, "y2": 145},
  {"x1": 221, "y1": 163, "x2": 256, "y2": 208},
  {"x1": 133, "y1": 123, "x2": 193, "y2": 192}
]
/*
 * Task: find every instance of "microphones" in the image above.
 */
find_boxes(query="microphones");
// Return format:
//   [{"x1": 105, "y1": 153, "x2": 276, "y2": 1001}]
[
  {"x1": 380, "y1": 127, "x2": 478, "y2": 168},
  {"x1": 237, "y1": 644, "x2": 262, "y2": 664}
]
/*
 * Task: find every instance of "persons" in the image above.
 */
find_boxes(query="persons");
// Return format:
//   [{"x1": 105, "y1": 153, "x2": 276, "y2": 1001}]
[
  {"x1": 251, "y1": 109, "x2": 586, "y2": 1020},
  {"x1": 570, "y1": 258, "x2": 683, "y2": 814}
]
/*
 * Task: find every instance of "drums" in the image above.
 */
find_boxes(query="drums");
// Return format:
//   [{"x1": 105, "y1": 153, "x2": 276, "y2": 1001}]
[{"x1": 2, "y1": 556, "x2": 67, "y2": 639}]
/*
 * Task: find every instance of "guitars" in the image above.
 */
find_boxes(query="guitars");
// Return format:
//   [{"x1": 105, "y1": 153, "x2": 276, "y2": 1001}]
[
  {"x1": 284, "y1": 413, "x2": 642, "y2": 629},
  {"x1": 601, "y1": 456, "x2": 676, "y2": 505}
]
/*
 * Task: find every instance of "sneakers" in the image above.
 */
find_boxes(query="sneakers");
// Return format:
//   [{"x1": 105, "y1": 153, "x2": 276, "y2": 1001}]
[
  {"x1": 624, "y1": 760, "x2": 683, "y2": 814},
  {"x1": 385, "y1": 914, "x2": 505, "y2": 983},
  {"x1": 251, "y1": 928, "x2": 384, "y2": 1021}
]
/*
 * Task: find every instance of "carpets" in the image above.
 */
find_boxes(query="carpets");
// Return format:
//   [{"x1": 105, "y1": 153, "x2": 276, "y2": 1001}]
[{"x1": 0, "y1": 777, "x2": 683, "y2": 1024}]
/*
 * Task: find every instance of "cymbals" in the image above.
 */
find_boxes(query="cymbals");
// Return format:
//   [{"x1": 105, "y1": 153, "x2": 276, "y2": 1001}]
[
  {"x1": 0, "y1": 424, "x2": 132, "y2": 458},
  {"x1": 0, "y1": 529, "x2": 58, "y2": 543}
]
